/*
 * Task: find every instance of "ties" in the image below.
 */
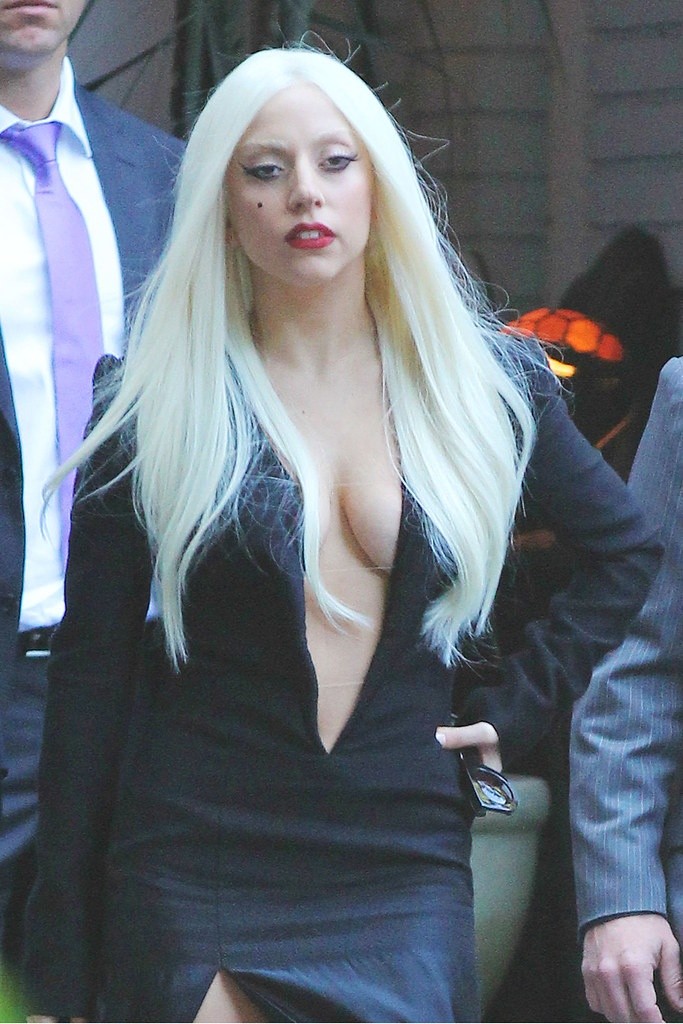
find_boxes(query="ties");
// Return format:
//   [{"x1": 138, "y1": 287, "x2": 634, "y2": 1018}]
[{"x1": 1, "y1": 119, "x2": 103, "y2": 576}]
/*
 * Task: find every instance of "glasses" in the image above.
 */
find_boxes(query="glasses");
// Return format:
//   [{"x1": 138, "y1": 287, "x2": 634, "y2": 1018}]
[{"x1": 448, "y1": 711, "x2": 518, "y2": 818}]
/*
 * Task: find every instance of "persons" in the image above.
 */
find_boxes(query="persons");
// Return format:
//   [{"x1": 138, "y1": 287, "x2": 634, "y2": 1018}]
[
  {"x1": 569, "y1": 355, "x2": 683, "y2": 1024},
  {"x1": 0, "y1": 0, "x2": 191, "y2": 973},
  {"x1": 23, "y1": 47, "x2": 666, "y2": 1024}
]
